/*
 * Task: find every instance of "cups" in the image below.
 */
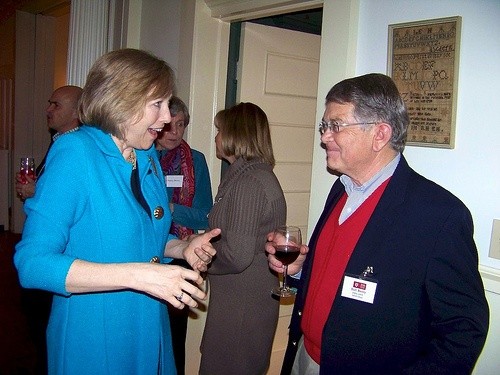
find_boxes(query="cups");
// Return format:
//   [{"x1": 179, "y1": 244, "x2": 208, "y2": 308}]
[{"x1": 20, "y1": 157, "x2": 35, "y2": 184}]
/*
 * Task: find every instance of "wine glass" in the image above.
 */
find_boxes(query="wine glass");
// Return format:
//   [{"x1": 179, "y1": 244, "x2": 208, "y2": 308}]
[{"x1": 270, "y1": 225, "x2": 302, "y2": 297}]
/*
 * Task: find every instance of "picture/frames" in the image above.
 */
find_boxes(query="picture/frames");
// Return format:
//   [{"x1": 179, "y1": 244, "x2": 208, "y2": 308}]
[{"x1": 387, "y1": 16, "x2": 462, "y2": 149}]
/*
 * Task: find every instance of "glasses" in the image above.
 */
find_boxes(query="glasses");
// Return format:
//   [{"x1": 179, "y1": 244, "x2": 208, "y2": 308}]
[{"x1": 317, "y1": 120, "x2": 381, "y2": 134}]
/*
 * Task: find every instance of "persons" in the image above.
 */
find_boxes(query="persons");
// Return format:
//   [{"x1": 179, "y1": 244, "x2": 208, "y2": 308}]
[
  {"x1": 197, "y1": 101, "x2": 287, "y2": 375},
  {"x1": 157, "y1": 96, "x2": 214, "y2": 375},
  {"x1": 15, "y1": 85, "x2": 86, "y2": 199},
  {"x1": 264, "y1": 72, "x2": 491, "y2": 375},
  {"x1": 13, "y1": 47, "x2": 221, "y2": 375}
]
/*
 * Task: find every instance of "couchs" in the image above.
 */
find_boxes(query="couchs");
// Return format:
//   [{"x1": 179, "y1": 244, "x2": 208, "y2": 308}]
[{"x1": 0, "y1": 230, "x2": 47, "y2": 375}]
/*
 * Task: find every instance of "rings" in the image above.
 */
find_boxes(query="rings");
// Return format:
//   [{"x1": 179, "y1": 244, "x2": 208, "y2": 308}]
[{"x1": 178, "y1": 292, "x2": 185, "y2": 302}]
